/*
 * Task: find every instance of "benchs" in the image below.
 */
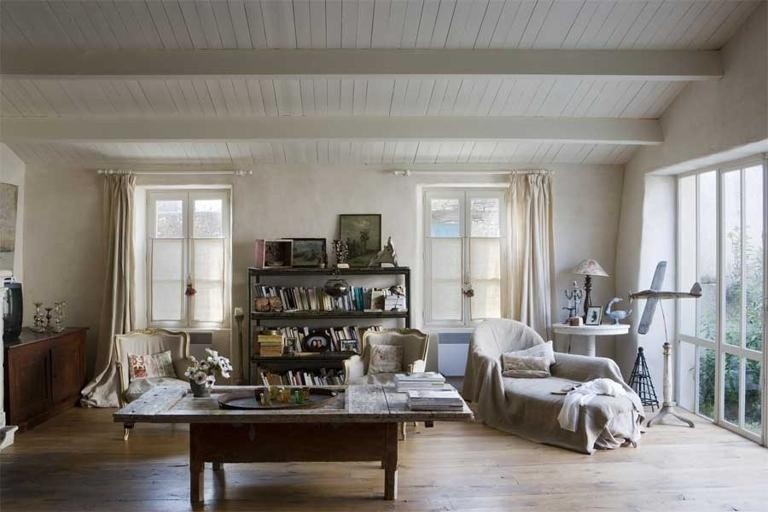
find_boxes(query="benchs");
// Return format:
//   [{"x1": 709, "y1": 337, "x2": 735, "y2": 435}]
[{"x1": 463, "y1": 318, "x2": 645, "y2": 456}]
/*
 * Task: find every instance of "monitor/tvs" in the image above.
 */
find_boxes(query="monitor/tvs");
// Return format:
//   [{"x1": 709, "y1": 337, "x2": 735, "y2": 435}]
[{"x1": 0, "y1": 283, "x2": 22, "y2": 341}]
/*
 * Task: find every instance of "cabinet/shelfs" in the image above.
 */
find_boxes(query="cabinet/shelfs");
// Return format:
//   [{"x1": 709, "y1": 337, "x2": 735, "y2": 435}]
[
  {"x1": 5, "y1": 326, "x2": 90, "y2": 433},
  {"x1": 247, "y1": 267, "x2": 410, "y2": 385}
]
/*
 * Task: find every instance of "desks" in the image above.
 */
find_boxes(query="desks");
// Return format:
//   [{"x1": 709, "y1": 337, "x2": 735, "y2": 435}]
[
  {"x1": 553, "y1": 323, "x2": 631, "y2": 357},
  {"x1": 112, "y1": 384, "x2": 474, "y2": 502}
]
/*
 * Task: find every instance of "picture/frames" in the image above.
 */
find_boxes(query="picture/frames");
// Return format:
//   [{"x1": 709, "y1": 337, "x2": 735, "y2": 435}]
[
  {"x1": 339, "y1": 214, "x2": 381, "y2": 267},
  {"x1": 585, "y1": 306, "x2": 603, "y2": 325},
  {"x1": 263, "y1": 239, "x2": 294, "y2": 269},
  {"x1": 281, "y1": 238, "x2": 326, "y2": 267}
]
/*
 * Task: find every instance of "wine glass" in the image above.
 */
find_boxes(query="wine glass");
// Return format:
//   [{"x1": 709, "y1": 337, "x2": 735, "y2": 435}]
[{"x1": 323, "y1": 279, "x2": 350, "y2": 313}]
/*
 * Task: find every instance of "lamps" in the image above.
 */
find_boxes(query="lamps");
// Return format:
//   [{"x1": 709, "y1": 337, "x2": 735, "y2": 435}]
[{"x1": 571, "y1": 258, "x2": 609, "y2": 324}]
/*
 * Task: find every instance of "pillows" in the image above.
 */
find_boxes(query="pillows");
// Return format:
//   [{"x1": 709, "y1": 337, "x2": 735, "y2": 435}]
[
  {"x1": 503, "y1": 353, "x2": 551, "y2": 378},
  {"x1": 507, "y1": 341, "x2": 556, "y2": 365},
  {"x1": 366, "y1": 343, "x2": 405, "y2": 375},
  {"x1": 128, "y1": 349, "x2": 177, "y2": 382}
]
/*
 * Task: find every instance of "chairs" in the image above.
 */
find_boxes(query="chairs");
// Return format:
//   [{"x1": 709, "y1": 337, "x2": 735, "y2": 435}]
[
  {"x1": 342, "y1": 328, "x2": 430, "y2": 440},
  {"x1": 115, "y1": 326, "x2": 199, "y2": 442}
]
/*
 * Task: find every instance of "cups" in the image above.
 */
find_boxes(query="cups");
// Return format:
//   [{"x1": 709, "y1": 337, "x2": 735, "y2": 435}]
[{"x1": 253, "y1": 385, "x2": 312, "y2": 406}]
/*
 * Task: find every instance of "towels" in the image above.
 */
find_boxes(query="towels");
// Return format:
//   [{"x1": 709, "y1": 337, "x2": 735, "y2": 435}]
[{"x1": 558, "y1": 377, "x2": 625, "y2": 432}]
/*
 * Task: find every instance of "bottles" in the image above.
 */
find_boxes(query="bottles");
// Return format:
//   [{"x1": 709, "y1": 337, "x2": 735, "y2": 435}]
[{"x1": 31, "y1": 300, "x2": 68, "y2": 335}]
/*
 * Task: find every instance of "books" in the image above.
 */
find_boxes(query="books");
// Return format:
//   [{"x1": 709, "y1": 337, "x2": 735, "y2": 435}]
[
  {"x1": 392, "y1": 368, "x2": 464, "y2": 412},
  {"x1": 253, "y1": 324, "x2": 384, "y2": 358},
  {"x1": 255, "y1": 364, "x2": 345, "y2": 385},
  {"x1": 253, "y1": 280, "x2": 406, "y2": 314}
]
